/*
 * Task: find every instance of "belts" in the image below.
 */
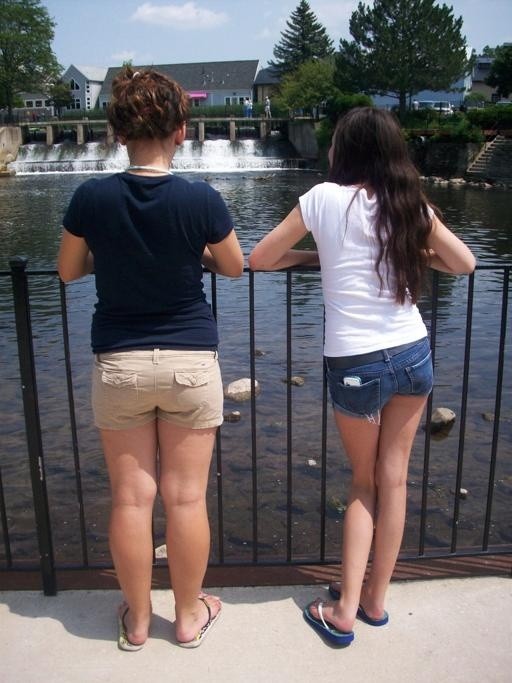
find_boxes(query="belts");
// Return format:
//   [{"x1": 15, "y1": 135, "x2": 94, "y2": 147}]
[{"x1": 327, "y1": 337, "x2": 425, "y2": 369}]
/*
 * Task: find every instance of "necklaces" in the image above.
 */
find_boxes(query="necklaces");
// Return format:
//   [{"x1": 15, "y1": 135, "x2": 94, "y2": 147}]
[{"x1": 124, "y1": 165, "x2": 174, "y2": 175}]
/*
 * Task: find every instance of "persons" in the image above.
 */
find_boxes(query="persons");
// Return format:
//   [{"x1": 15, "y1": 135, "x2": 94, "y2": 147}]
[
  {"x1": 412, "y1": 99, "x2": 420, "y2": 112},
  {"x1": 243, "y1": 96, "x2": 250, "y2": 119},
  {"x1": 247, "y1": 100, "x2": 253, "y2": 120},
  {"x1": 264, "y1": 96, "x2": 271, "y2": 120},
  {"x1": 247, "y1": 105, "x2": 476, "y2": 645},
  {"x1": 57, "y1": 66, "x2": 245, "y2": 653}
]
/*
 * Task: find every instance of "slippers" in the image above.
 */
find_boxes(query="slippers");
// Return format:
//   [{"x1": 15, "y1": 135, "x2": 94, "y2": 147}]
[
  {"x1": 177, "y1": 592, "x2": 223, "y2": 648},
  {"x1": 117, "y1": 601, "x2": 147, "y2": 652},
  {"x1": 304, "y1": 597, "x2": 354, "y2": 645},
  {"x1": 329, "y1": 579, "x2": 389, "y2": 626}
]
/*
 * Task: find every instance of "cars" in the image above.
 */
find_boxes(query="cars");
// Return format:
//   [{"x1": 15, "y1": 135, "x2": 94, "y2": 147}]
[{"x1": 417, "y1": 101, "x2": 453, "y2": 114}]
